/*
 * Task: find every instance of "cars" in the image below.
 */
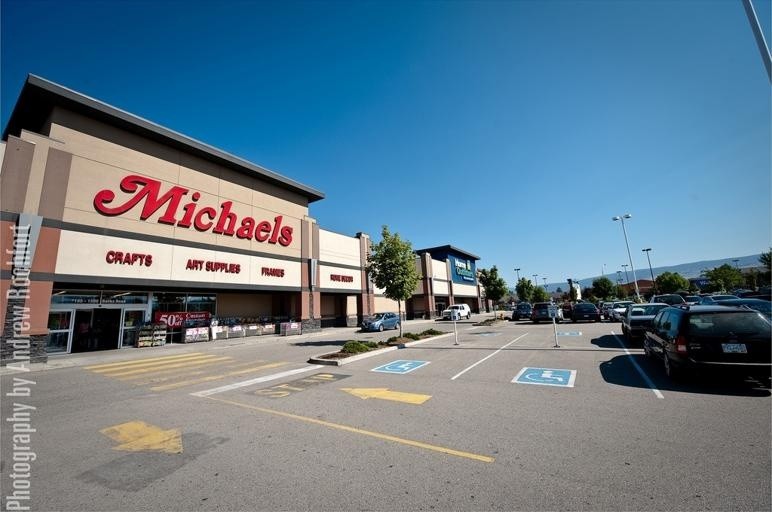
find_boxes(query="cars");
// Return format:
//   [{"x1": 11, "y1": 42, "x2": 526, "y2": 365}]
[
  {"x1": 512, "y1": 288, "x2": 772, "y2": 382},
  {"x1": 361, "y1": 312, "x2": 400, "y2": 332}
]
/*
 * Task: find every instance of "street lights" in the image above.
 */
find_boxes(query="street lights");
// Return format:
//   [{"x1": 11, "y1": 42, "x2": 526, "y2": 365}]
[
  {"x1": 513, "y1": 268, "x2": 520, "y2": 281},
  {"x1": 542, "y1": 278, "x2": 548, "y2": 292},
  {"x1": 612, "y1": 214, "x2": 640, "y2": 303},
  {"x1": 732, "y1": 259, "x2": 740, "y2": 269},
  {"x1": 642, "y1": 248, "x2": 655, "y2": 294},
  {"x1": 532, "y1": 273, "x2": 538, "y2": 288},
  {"x1": 602, "y1": 263, "x2": 606, "y2": 276}
]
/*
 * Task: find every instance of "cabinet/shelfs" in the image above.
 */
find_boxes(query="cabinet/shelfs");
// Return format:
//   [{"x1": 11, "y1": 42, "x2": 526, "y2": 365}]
[{"x1": 135, "y1": 321, "x2": 167, "y2": 348}]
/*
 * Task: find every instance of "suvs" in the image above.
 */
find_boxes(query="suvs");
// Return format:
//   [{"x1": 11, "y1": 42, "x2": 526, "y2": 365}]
[{"x1": 443, "y1": 304, "x2": 471, "y2": 320}]
